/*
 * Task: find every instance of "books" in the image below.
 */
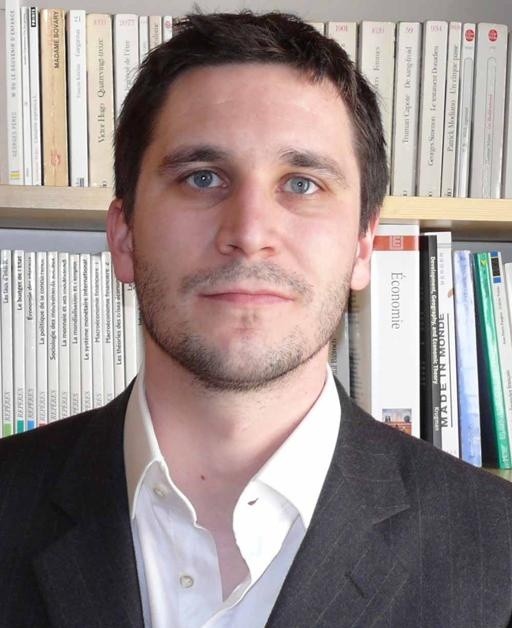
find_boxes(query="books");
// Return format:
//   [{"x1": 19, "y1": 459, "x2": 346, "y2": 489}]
[
  {"x1": 0, "y1": 1, "x2": 200, "y2": 443},
  {"x1": 301, "y1": 19, "x2": 510, "y2": 470}
]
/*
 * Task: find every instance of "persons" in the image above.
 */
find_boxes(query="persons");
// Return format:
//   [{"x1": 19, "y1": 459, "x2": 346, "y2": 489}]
[{"x1": 0, "y1": 3, "x2": 512, "y2": 628}]
